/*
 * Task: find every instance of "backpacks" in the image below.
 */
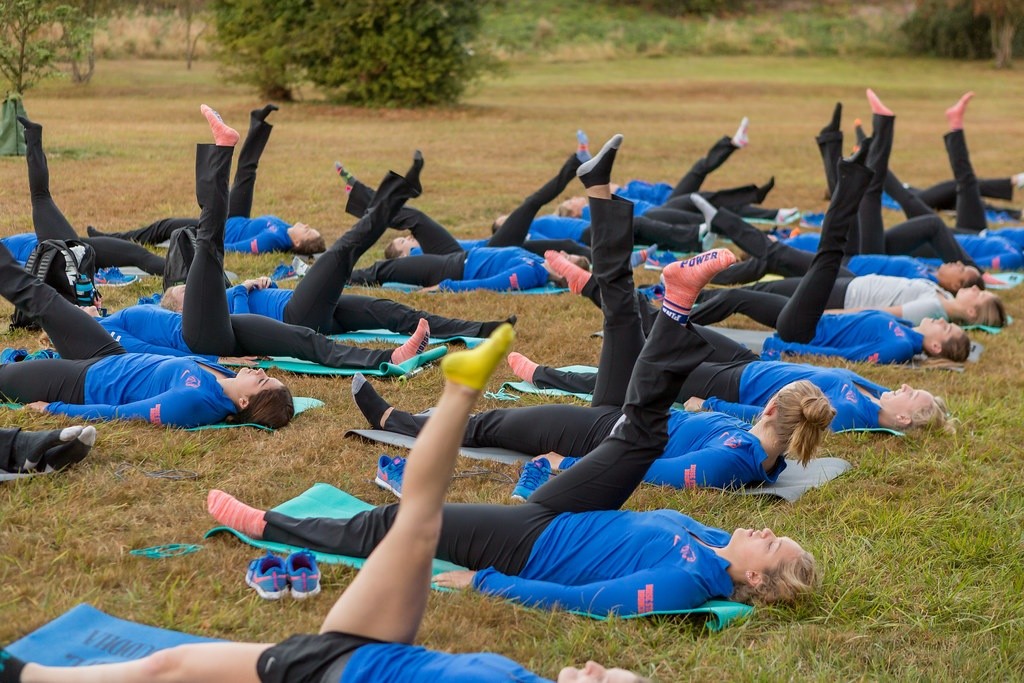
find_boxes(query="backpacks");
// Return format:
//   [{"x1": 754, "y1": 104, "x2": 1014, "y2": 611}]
[
  {"x1": 163, "y1": 225, "x2": 233, "y2": 295},
  {"x1": 9, "y1": 238, "x2": 102, "y2": 336}
]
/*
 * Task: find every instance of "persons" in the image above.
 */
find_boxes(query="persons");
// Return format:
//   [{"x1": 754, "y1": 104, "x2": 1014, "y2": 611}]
[{"x1": 0, "y1": 88, "x2": 1024, "y2": 683}]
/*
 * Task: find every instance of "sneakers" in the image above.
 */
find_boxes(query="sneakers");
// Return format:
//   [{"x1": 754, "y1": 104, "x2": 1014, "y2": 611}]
[
  {"x1": 759, "y1": 337, "x2": 785, "y2": 360},
  {"x1": 0, "y1": 347, "x2": 59, "y2": 364},
  {"x1": 374, "y1": 455, "x2": 407, "y2": 498},
  {"x1": 767, "y1": 226, "x2": 791, "y2": 239},
  {"x1": 137, "y1": 293, "x2": 161, "y2": 305},
  {"x1": 245, "y1": 547, "x2": 321, "y2": 599},
  {"x1": 644, "y1": 250, "x2": 679, "y2": 271},
  {"x1": 94, "y1": 266, "x2": 138, "y2": 287},
  {"x1": 985, "y1": 209, "x2": 1013, "y2": 221},
  {"x1": 799, "y1": 211, "x2": 825, "y2": 228},
  {"x1": 635, "y1": 283, "x2": 665, "y2": 306},
  {"x1": 511, "y1": 458, "x2": 557, "y2": 502},
  {"x1": 267, "y1": 264, "x2": 298, "y2": 283}
]
